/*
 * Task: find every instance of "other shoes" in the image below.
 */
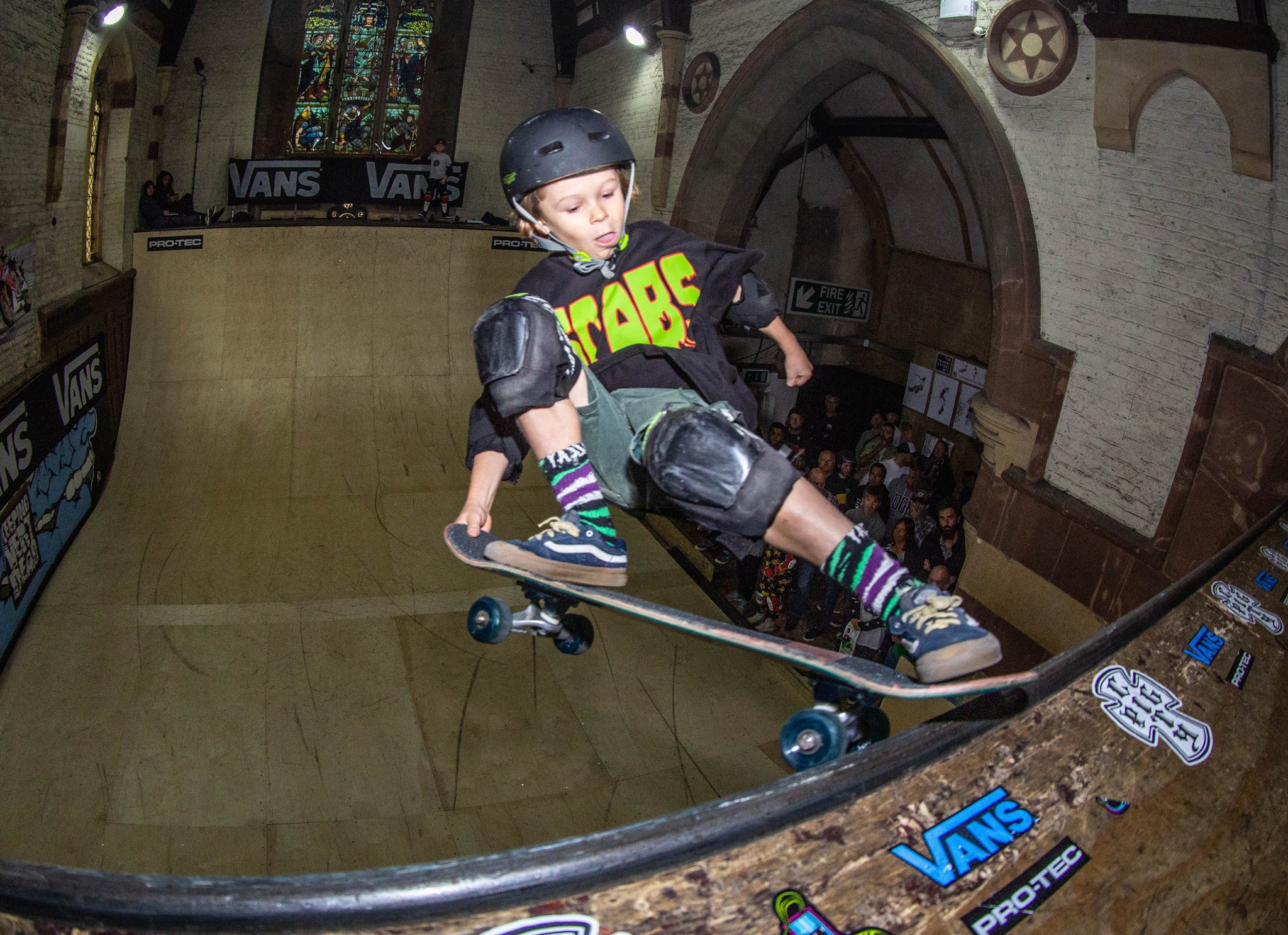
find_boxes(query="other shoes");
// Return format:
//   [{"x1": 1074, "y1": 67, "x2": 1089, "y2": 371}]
[
  {"x1": 785, "y1": 617, "x2": 800, "y2": 630},
  {"x1": 442, "y1": 212, "x2": 451, "y2": 219},
  {"x1": 419, "y1": 212, "x2": 427, "y2": 217},
  {"x1": 213, "y1": 207, "x2": 225, "y2": 224},
  {"x1": 737, "y1": 601, "x2": 751, "y2": 615},
  {"x1": 747, "y1": 612, "x2": 766, "y2": 623},
  {"x1": 211, "y1": 206, "x2": 217, "y2": 220},
  {"x1": 206, "y1": 208, "x2": 213, "y2": 225},
  {"x1": 755, "y1": 621, "x2": 774, "y2": 633},
  {"x1": 802, "y1": 626, "x2": 824, "y2": 641}
]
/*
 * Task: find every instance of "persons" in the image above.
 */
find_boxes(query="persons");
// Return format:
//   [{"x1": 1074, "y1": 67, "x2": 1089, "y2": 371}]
[
  {"x1": 411, "y1": 137, "x2": 452, "y2": 219},
  {"x1": 450, "y1": 105, "x2": 1002, "y2": 686},
  {"x1": 139, "y1": 170, "x2": 216, "y2": 230},
  {"x1": 694, "y1": 389, "x2": 981, "y2": 668}
]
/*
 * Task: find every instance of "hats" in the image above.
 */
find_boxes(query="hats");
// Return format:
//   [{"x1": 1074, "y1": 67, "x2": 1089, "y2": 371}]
[
  {"x1": 788, "y1": 408, "x2": 808, "y2": 422},
  {"x1": 897, "y1": 443, "x2": 919, "y2": 456}
]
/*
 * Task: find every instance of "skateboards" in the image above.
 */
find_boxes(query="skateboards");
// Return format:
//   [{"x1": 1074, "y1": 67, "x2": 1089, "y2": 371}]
[
  {"x1": 407, "y1": 215, "x2": 458, "y2": 224},
  {"x1": 444, "y1": 525, "x2": 1039, "y2": 772},
  {"x1": 838, "y1": 619, "x2": 861, "y2": 657}
]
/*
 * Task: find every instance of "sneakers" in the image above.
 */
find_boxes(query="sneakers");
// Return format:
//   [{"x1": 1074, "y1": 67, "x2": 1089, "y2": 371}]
[
  {"x1": 483, "y1": 510, "x2": 628, "y2": 588},
  {"x1": 696, "y1": 538, "x2": 717, "y2": 551},
  {"x1": 887, "y1": 581, "x2": 1002, "y2": 684},
  {"x1": 716, "y1": 551, "x2": 734, "y2": 565}
]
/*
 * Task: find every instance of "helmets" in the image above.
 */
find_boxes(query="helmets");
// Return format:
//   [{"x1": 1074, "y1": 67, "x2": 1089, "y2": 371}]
[
  {"x1": 500, "y1": 108, "x2": 636, "y2": 210},
  {"x1": 435, "y1": 138, "x2": 447, "y2": 146}
]
